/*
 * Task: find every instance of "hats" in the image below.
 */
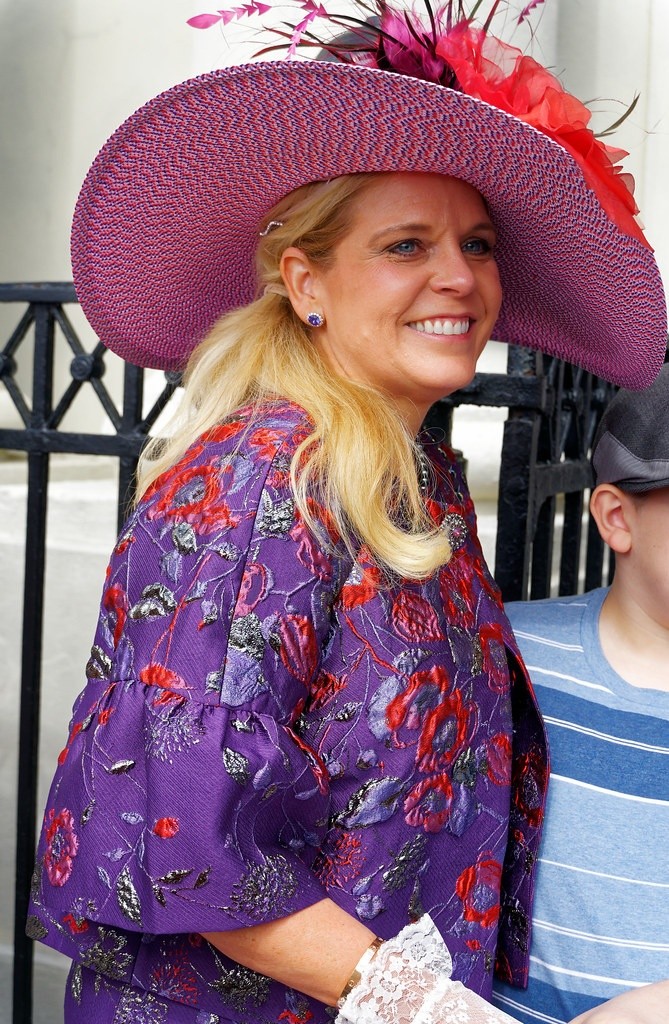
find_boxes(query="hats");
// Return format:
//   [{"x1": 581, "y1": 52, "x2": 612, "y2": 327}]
[
  {"x1": 70, "y1": 0, "x2": 668, "y2": 392},
  {"x1": 590, "y1": 364, "x2": 669, "y2": 497}
]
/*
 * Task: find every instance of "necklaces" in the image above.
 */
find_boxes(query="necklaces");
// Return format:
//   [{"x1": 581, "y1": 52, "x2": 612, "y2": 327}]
[{"x1": 411, "y1": 428, "x2": 469, "y2": 553}]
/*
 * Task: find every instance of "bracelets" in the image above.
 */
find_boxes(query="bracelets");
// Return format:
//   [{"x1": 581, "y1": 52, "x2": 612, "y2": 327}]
[{"x1": 337, "y1": 937, "x2": 385, "y2": 1002}]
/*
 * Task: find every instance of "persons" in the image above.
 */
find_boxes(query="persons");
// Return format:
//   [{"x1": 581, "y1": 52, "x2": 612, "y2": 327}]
[
  {"x1": 25, "y1": 170, "x2": 552, "y2": 1024},
  {"x1": 491, "y1": 366, "x2": 669, "y2": 1024}
]
